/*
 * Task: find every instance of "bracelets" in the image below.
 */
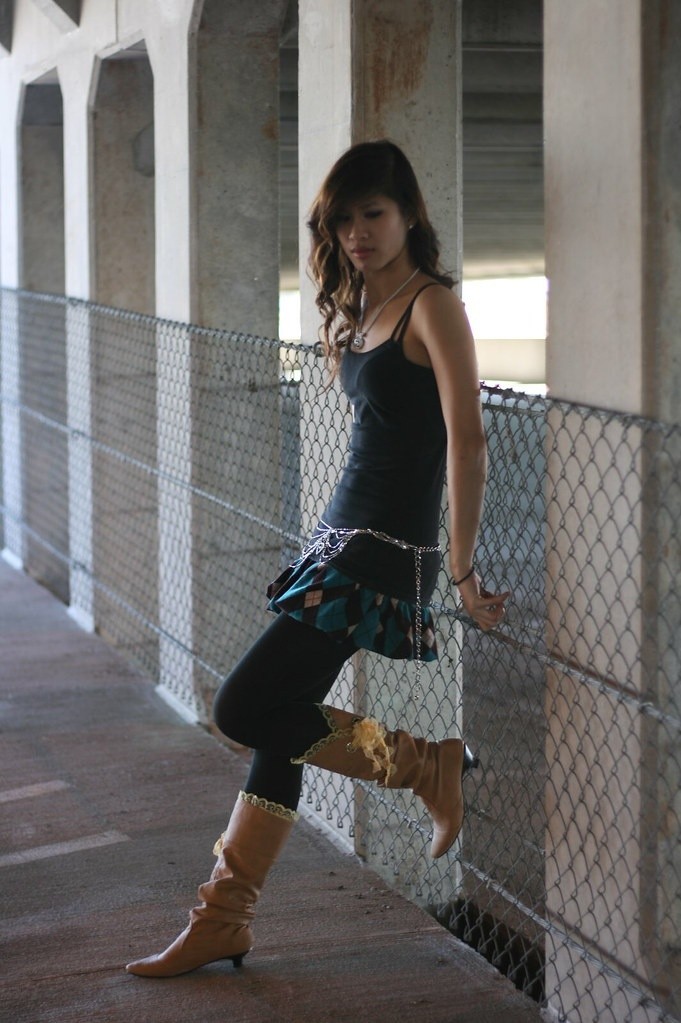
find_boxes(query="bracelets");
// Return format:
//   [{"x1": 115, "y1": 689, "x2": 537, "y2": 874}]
[{"x1": 453, "y1": 566, "x2": 475, "y2": 585}]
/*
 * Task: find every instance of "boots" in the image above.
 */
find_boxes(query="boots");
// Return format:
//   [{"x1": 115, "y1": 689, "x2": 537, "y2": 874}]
[
  {"x1": 125, "y1": 789, "x2": 300, "y2": 978},
  {"x1": 291, "y1": 703, "x2": 480, "y2": 858}
]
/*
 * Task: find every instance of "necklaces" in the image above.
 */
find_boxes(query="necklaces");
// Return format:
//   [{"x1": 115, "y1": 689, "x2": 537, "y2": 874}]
[{"x1": 354, "y1": 266, "x2": 421, "y2": 349}]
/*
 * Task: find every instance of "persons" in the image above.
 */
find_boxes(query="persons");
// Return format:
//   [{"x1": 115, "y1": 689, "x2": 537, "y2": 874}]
[{"x1": 127, "y1": 140, "x2": 511, "y2": 978}]
